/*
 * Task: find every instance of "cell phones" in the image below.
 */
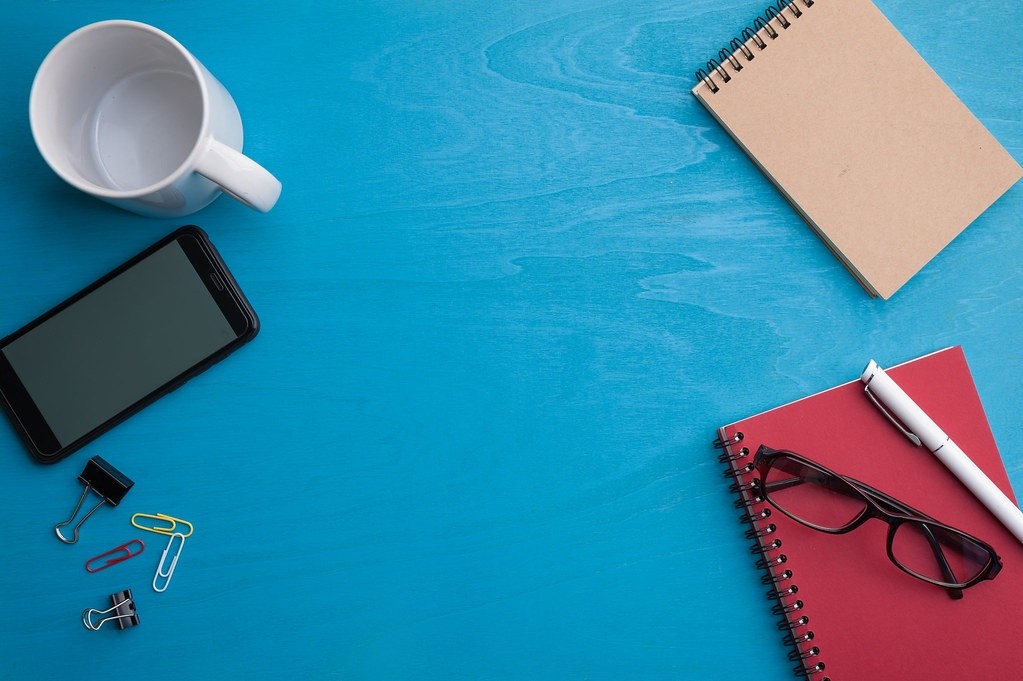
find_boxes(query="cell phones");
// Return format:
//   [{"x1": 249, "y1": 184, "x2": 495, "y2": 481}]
[{"x1": 0, "y1": 225, "x2": 259, "y2": 466}]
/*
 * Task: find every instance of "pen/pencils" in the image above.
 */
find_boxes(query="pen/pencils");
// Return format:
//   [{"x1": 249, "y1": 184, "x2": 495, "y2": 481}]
[{"x1": 860, "y1": 359, "x2": 1022, "y2": 544}]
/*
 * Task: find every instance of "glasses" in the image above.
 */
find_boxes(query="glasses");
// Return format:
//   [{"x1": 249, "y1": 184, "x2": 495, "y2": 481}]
[{"x1": 751, "y1": 443, "x2": 1003, "y2": 600}]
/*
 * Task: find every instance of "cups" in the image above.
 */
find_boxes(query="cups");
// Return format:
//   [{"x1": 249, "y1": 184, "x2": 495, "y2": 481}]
[{"x1": 28, "y1": 20, "x2": 282, "y2": 217}]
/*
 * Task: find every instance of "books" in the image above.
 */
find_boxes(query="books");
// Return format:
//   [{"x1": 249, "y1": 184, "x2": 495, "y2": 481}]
[
  {"x1": 714, "y1": 344, "x2": 1022, "y2": 681},
  {"x1": 690, "y1": 0, "x2": 1023, "y2": 300}
]
